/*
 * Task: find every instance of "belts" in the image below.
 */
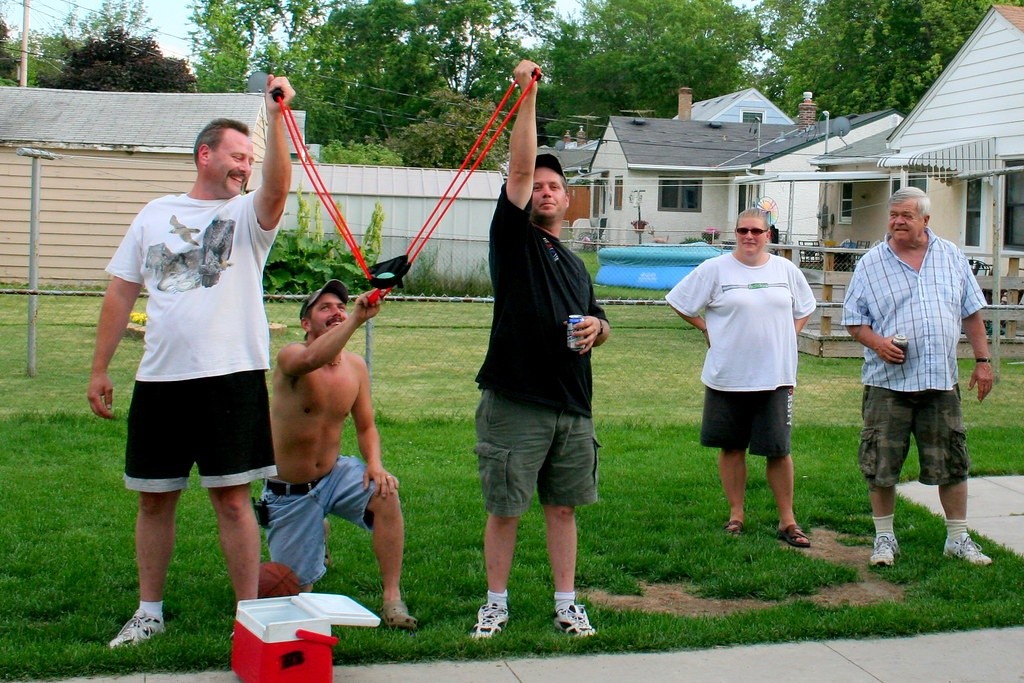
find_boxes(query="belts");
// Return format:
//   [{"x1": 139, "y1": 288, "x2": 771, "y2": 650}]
[{"x1": 267, "y1": 472, "x2": 329, "y2": 497}]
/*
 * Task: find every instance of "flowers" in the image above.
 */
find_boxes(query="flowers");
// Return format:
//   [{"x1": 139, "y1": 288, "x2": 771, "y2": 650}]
[{"x1": 631, "y1": 220, "x2": 648, "y2": 226}]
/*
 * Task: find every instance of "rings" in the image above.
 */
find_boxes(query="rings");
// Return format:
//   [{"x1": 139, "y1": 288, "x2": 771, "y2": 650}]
[{"x1": 287, "y1": 85, "x2": 290, "y2": 87}]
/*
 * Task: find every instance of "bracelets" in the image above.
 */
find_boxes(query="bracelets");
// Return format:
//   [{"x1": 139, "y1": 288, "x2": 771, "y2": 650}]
[
  {"x1": 597, "y1": 320, "x2": 603, "y2": 336},
  {"x1": 976, "y1": 358, "x2": 991, "y2": 363},
  {"x1": 701, "y1": 329, "x2": 707, "y2": 333}
]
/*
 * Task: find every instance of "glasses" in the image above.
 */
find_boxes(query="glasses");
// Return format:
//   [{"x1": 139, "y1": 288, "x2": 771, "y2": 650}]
[{"x1": 736, "y1": 227, "x2": 769, "y2": 237}]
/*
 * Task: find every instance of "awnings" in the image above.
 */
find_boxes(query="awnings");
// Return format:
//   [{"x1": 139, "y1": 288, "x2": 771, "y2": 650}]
[
  {"x1": 734, "y1": 171, "x2": 890, "y2": 244},
  {"x1": 568, "y1": 170, "x2": 610, "y2": 185},
  {"x1": 876, "y1": 137, "x2": 996, "y2": 186}
]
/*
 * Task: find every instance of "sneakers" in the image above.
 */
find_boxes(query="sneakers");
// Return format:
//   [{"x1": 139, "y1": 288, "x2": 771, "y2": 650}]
[
  {"x1": 554, "y1": 603, "x2": 597, "y2": 638},
  {"x1": 107, "y1": 608, "x2": 167, "y2": 650},
  {"x1": 942, "y1": 533, "x2": 992, "y2": 567},
  {"x1": 869, "y1": 534, "x2": 901, "y2": 566},
  {"x1": 470, "y1": 603, "x2": 510, "y2": 641}
]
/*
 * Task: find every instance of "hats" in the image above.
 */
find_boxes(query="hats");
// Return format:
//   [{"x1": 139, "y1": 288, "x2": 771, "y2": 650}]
[
  {"x1": 299, "y1": 279, "x2": 349, "y2": 319},
  {"x1": 535, "y1": 153, "x2": 568, "y2": 181}
]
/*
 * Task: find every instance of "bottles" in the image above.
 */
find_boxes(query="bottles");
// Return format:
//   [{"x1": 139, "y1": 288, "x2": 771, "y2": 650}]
[{"x1": 893, "y1": 334, "x2": 907, "y2": 346}]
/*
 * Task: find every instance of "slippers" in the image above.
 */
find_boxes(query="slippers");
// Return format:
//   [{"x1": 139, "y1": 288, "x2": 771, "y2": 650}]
[
  {"x1": 776, "y1": 525, "x2": 810, "y2": 547},
  {"x1": 722, "y1": 520, "x2": 747, "y2": 535},
  {"x1": 379, "y1": 600, "x2": 419, "y2": 629}
]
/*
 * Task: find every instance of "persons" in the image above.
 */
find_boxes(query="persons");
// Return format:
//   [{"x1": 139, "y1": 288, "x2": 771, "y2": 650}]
[
  {"x1": 261, "y1": 279, "x2": 417, "y2": 629},
  {"x1": 470, "y1": 60, "x2": 611, "y2": 640},
  {"x1": 665, "y1": 208, "x2": 816, "y2": 547},
  {"x1": 88, "y1": 74, "x2": 296, "y2": 648},
  {"x1": 841, "y1": 186, "x2": 994, "y2": 569}
]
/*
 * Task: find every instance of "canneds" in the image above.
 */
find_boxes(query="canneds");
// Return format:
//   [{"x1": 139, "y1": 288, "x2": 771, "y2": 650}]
[
  {"x1": 567, "y1": 315, "x2": 586, "y2": 352},
  {"x1": 891, "y1": 334, "x2": 909, "y2": 363}
]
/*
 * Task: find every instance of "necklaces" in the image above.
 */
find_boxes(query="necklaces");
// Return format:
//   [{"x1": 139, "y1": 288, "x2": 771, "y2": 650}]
[{"x1": 328, "y1": 356, "x2": 342, "y2": 366}]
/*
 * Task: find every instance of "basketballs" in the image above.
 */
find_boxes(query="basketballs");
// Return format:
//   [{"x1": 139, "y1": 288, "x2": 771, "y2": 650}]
[{"x1": 258, "y1": 561, "x2": 297, "y2": 599}]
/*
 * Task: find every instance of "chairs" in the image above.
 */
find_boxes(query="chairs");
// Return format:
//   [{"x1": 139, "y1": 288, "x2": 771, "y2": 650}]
[
  {"x1": 856, "y1": 241, "x2": 870, "y2": 260},
  {"x1": 840, "y1": 239, "x2": 851, "y2": 246},
  {"x1": 560, "y1": 218, "x2": 607, "y2": 252},
  {"x1": 799, "y1": 240, "x2": 822, "y2": 263}
]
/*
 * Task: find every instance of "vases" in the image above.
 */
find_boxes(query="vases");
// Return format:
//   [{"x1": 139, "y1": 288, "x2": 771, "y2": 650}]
[{"x1": 634, "y1": 224, "x2": 644, "y2": 233}]
